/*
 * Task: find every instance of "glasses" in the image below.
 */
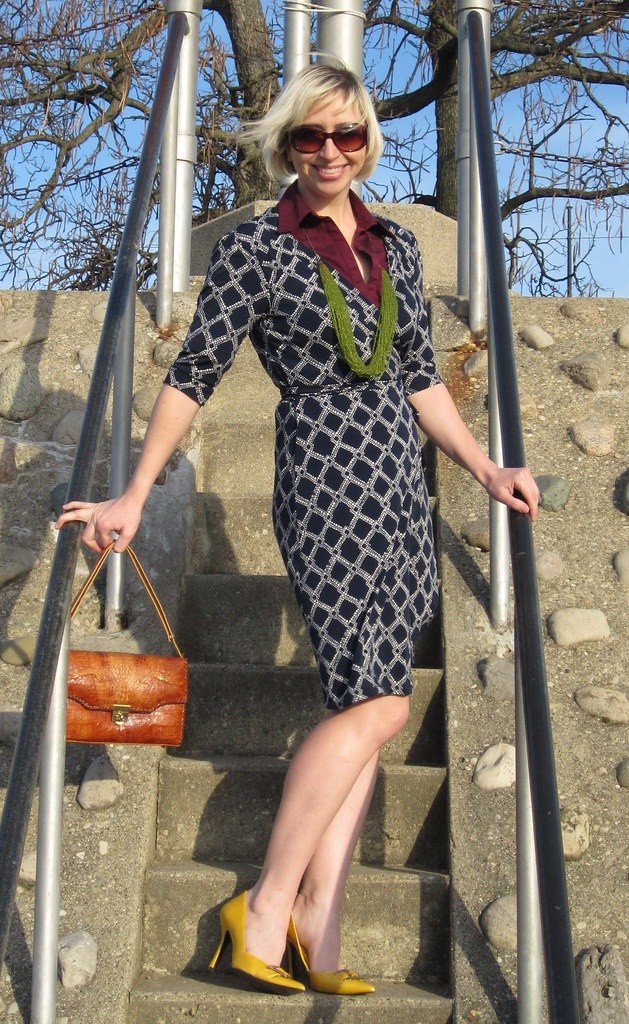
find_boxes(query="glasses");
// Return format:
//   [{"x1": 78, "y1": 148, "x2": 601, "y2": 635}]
[{"x1": 285, "y1": 123, "x2": 369, "y2": 153}]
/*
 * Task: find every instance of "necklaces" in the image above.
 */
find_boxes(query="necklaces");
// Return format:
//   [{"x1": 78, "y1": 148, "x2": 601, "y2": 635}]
[{"x1": 300, "y1": 225, "x2": 396, "y2": 379}]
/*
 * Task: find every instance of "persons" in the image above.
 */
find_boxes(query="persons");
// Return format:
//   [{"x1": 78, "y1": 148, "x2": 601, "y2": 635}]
[{"x1": 56, "y1": 63, "x2": 539, "y2": 995}]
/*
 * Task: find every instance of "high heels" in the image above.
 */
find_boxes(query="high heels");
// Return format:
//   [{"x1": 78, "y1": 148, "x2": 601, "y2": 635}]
[
  {"x1": 285, "y1": 912, "x2": 376, "y2": 995},
  {"x1": 208, "y1": 891, "x2": 306, "y2": 995}
]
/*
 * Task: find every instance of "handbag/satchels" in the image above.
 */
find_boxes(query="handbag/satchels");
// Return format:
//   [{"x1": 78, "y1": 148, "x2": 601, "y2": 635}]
[{"x1": 66, "y1": 541, "x2": 189, "y2": 747}]
